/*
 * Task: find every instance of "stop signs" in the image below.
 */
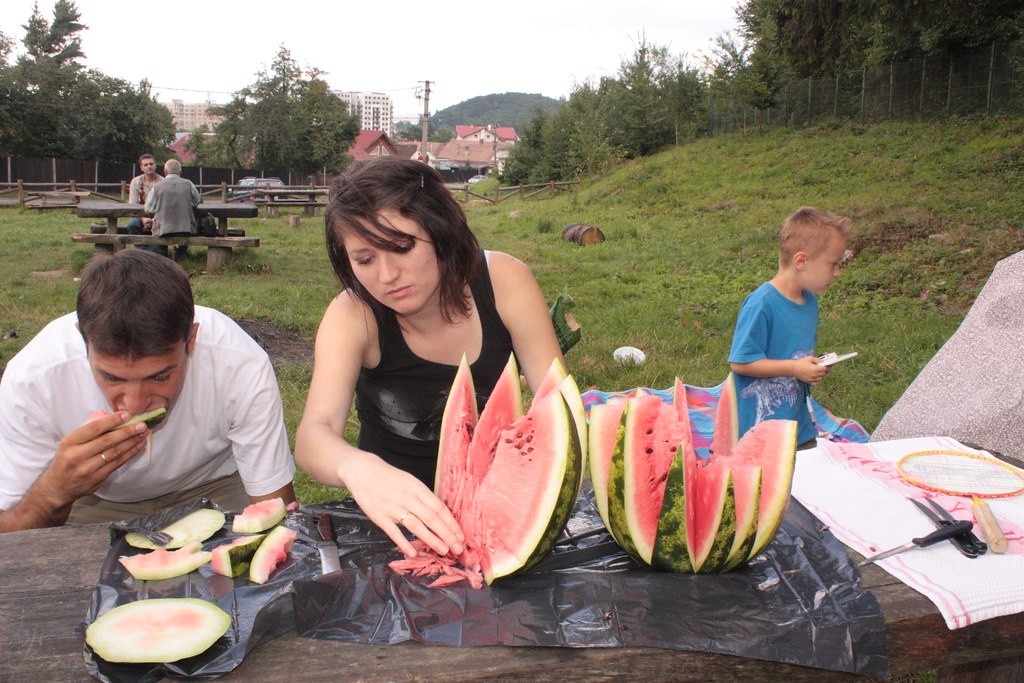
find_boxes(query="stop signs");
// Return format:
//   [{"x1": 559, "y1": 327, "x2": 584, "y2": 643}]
[{"x1": 418, "y1": 157, "x2": 424, "y2": 162}]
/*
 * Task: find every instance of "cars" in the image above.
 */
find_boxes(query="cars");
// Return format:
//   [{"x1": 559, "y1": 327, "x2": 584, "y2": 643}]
[
  {"x1": 227, "y1": 177, "x2": 256, "y2": 198},
  {"x1": 468, "y1": 175, "x2": 488, "y2": 183},
  {"x1": 250, "y1": 178, "x2": 285, "y2": 200}
]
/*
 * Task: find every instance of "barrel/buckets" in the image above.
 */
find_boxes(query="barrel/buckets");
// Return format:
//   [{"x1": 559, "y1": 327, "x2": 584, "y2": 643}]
[{"x1": 562, "y1": 224, "x2": 605, "y2": 244}]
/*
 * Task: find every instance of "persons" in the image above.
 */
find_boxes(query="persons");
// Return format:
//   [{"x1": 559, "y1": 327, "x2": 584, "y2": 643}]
[
  {"x1": 294, "y1": 158, "x2": 569, "y2": 558},
  {"x1": 1, "y1": 249, "x2": 296, "y2": 532},
  {"x1": 127, "y1": 154, "x2": 165, "y2": 235},
  {"x1": 729, "y1": 207, "x2": 850, "y2": 450},
  {"x1": 145, "y1": 159, "x2": 202, "y2": 262}
]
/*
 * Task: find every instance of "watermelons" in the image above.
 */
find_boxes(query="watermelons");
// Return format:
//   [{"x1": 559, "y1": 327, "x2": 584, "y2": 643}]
[{"x1": 79, "y1": 352, "x2": 798, "y2": 678}]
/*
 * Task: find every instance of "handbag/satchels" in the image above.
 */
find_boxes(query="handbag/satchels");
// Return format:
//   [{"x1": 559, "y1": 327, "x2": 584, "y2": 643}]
[{"x1": 196, "y1": 212, "x2": 216, "y2": 237}]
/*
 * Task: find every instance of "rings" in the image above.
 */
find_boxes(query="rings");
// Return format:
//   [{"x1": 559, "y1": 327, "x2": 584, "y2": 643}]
[
  {"x1": 100, "y1": 452, "x2": 108, "y2": 463},
  {"x1": 400, "y1": 512, "x2": 410, "y2": 522}
]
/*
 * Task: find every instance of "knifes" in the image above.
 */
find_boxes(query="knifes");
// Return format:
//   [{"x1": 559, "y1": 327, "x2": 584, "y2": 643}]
[
  {"x1": 907, "y1": 496, "x2": 988, "y2": 558},
  {"x1": 314, "y1": 513, "x2": 342, "y2": 574},
  {"x1": 857, "y1": 520, "x2": 973, "y2": 568}
]
